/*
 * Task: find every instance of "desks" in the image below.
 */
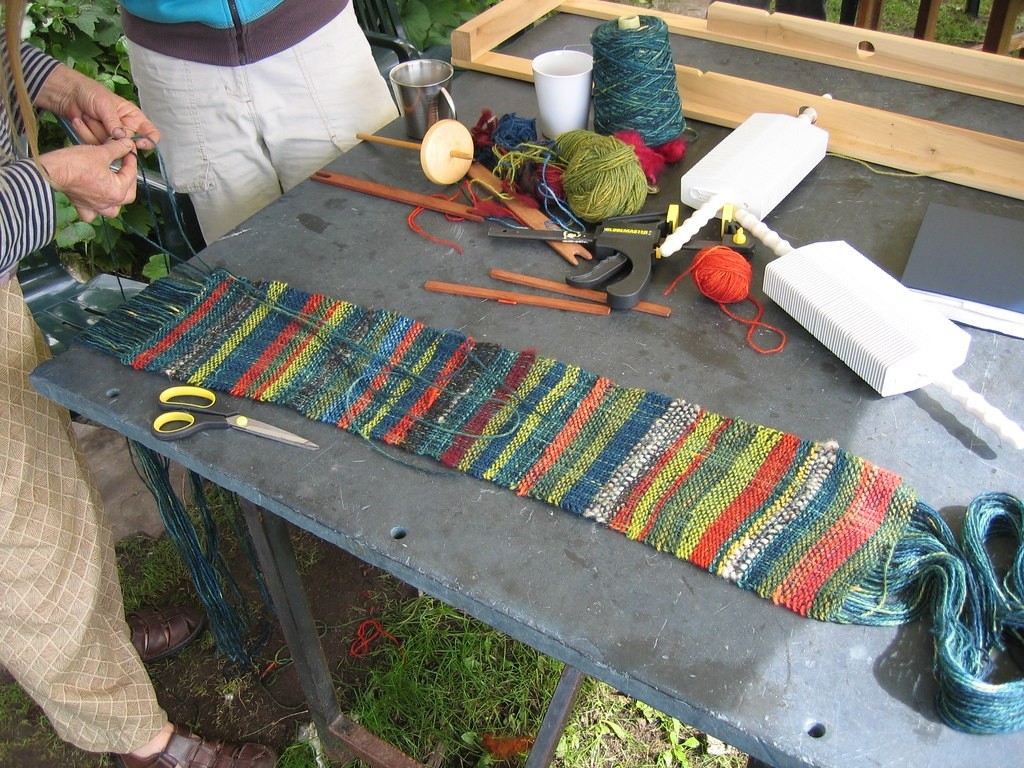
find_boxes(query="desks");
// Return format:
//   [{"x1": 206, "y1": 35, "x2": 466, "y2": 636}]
[{"x1": 22, "y1": 13, "x2": 1024, "y2": 768}]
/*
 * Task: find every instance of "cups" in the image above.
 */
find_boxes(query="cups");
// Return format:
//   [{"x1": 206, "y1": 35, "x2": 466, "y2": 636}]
[
  {"x1": 531, "y1": 49, "x2": 593, "y2": 140},
  {"x1": 389, "y1": 59, "x2": 457, "y2": 141}
]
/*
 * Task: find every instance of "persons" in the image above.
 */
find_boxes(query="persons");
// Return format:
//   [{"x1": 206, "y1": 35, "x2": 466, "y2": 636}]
[
  {"x1": 120, "y1": 0, "x2": 400, "y2": 247},
  {"x1": 0, "y1": 1, "x2": 281, "y2": 768}
]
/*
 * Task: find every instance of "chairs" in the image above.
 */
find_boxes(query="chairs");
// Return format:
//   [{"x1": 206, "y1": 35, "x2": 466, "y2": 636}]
[
  {"x1": 353, "y1": 0, "x2": 466, "y2": 112},
  {"x1": 18, "y1": 109, "x2": 210, "y2": 361}
]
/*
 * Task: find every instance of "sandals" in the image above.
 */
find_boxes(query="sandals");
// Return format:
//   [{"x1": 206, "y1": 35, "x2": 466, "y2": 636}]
[
  {"x1": 123, "y1": 603, "x2": 203, "y2": 663},
  {"x1": 110, "y1": 720, "x2": 278, "y2": 768}
]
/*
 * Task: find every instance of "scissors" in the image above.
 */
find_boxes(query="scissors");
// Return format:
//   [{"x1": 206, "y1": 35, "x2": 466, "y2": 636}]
[{"x1": 148, "y1": 385, "x2": 321, "y2": 452}]
